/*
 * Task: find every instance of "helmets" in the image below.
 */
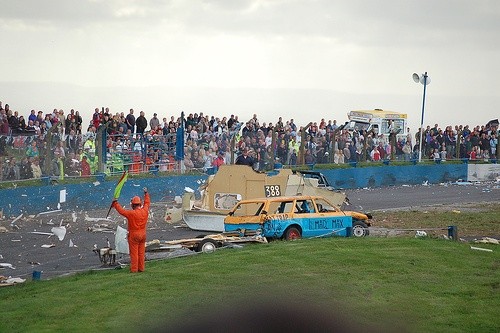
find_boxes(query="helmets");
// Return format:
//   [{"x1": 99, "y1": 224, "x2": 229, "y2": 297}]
[{"x1": 131, "y1": 196, "x2": 141, "y2": 204}]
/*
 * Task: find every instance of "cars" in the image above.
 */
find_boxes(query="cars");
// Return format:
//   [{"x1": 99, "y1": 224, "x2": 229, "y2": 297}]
[
  {"x1": 223, "y1": 197, "x2": 373, "y2": 242},
  {"x1": 290, "y1": 169, "x2": 335, "y2": 191}
]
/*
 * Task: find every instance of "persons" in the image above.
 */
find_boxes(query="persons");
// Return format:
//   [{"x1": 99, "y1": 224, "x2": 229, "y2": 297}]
[
  {"x1": 0, "y1": 108, "x2": 500, "y2": 178},
  {"x1": 113, "y1": 187, "x2": 150, "y2": 272}
]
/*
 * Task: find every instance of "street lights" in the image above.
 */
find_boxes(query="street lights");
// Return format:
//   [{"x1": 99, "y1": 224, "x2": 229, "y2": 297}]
[{"x1": 412, "y1": 71, "x2": 432, "y2": 163}]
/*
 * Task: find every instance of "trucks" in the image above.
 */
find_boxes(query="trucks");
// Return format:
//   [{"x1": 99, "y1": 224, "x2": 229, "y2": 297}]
[{"x1": 341, "y1": 109, "x2": 408, "y2": 144}]
